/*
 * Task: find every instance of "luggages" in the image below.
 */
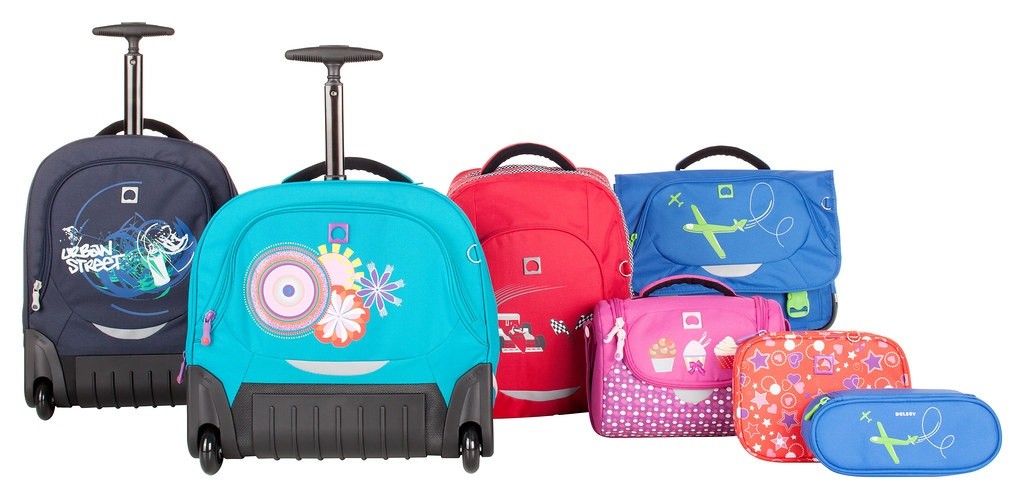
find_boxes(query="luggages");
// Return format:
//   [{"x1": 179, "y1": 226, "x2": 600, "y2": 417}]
[
  {"x1": 178, "y1": 41, "x2": 496, "y2": 478},
  {"x1": 23, "y1": 22, "x2": 241, "y2": 419}
]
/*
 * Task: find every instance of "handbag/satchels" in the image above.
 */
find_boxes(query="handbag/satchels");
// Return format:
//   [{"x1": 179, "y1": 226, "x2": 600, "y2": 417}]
[
  {"x1": 613, "y1": 144, "x2": 845, "y2": 334},
  {"x1": 446, "y1": 141, "x2": 637, "y2": 418},
  {"x1": 801, "y1": 388, "x2": 1006, "y2": 477},
  {"x1": 571, "y1": 273, "x2": 793, "y2": 439},
  {"x1": 732, "y1": 328, "x2": 911, "y2": 465}
]
